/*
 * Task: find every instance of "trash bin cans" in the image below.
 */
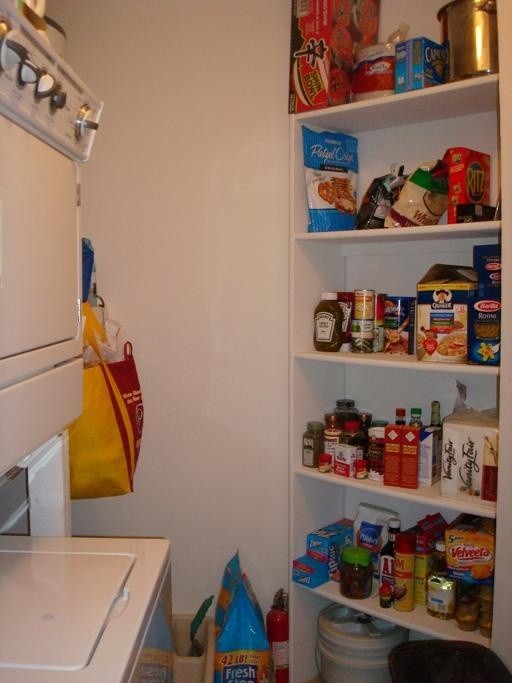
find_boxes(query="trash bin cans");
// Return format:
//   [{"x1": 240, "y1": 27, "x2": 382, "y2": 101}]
[{"x1": 388, "y1": 639, "x2": 512, "y2": 683}]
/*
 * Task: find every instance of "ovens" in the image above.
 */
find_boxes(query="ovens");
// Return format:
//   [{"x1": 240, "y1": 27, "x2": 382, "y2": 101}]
[{"x1": 2, "y1": 1, "x2": 179, "y2": 683}]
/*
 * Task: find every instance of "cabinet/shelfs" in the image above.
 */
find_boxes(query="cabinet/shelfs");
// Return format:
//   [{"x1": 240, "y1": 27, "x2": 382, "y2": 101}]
[{"x1": 282, "y1": 0, "x2": 512, "y2": 683}]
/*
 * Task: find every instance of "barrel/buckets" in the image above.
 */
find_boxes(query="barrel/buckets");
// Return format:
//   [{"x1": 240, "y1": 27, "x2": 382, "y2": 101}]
[{"x1": 312, "y1": 605, "x2": 407, "y2": 683}]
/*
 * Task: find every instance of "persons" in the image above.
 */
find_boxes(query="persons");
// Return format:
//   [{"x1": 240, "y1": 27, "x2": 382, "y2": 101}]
[{"x1": 435, "y1": 288, "x2": 449, "y2": 303}]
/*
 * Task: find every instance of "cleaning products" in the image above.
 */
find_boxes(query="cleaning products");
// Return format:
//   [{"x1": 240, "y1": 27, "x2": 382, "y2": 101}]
[{"x1": 216, "y1": 547, "x2": 273, "y2": 681}]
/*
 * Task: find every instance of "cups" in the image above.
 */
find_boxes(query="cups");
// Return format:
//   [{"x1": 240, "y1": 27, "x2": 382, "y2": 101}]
[
  {"x1": 455, "y1": 594, "x2": 478, "y2": 631},
  {"x1": 478, "y1": 590, "x2": 493, "y2": 639}
]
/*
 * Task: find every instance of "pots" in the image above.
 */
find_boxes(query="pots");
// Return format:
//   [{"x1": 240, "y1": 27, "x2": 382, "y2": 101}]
[{"x1": 435, "y1": 0, "x2": 498, "y2": 83}]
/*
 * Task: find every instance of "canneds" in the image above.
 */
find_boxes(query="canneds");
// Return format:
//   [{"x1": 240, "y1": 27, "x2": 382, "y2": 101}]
[
  {"x1": 351, "y1": 289, "x2": 387, "y2": 352},
  {"x1": 323, "y1": 430, "x2": 341, "y2": 468}
]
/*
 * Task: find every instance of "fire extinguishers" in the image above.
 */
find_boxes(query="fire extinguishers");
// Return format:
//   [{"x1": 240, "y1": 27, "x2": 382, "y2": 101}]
[{"x1": 266, "y1": 588, "x2": 290, "y2": 683}]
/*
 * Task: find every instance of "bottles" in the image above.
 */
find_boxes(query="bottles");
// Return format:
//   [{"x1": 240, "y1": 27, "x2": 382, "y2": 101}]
[
  {"x1": 432, "y1": 540, "x2": 448, "y2": 577},
  {"x1": 380, "y1": 517, "x2": 416, "y2": 612},
  {"x1": 312, "y1": 290, "x2": 343, "y2": 353},
  {"x1": 301, "y1": 397, "x2": 442, "y2": 479}
]
